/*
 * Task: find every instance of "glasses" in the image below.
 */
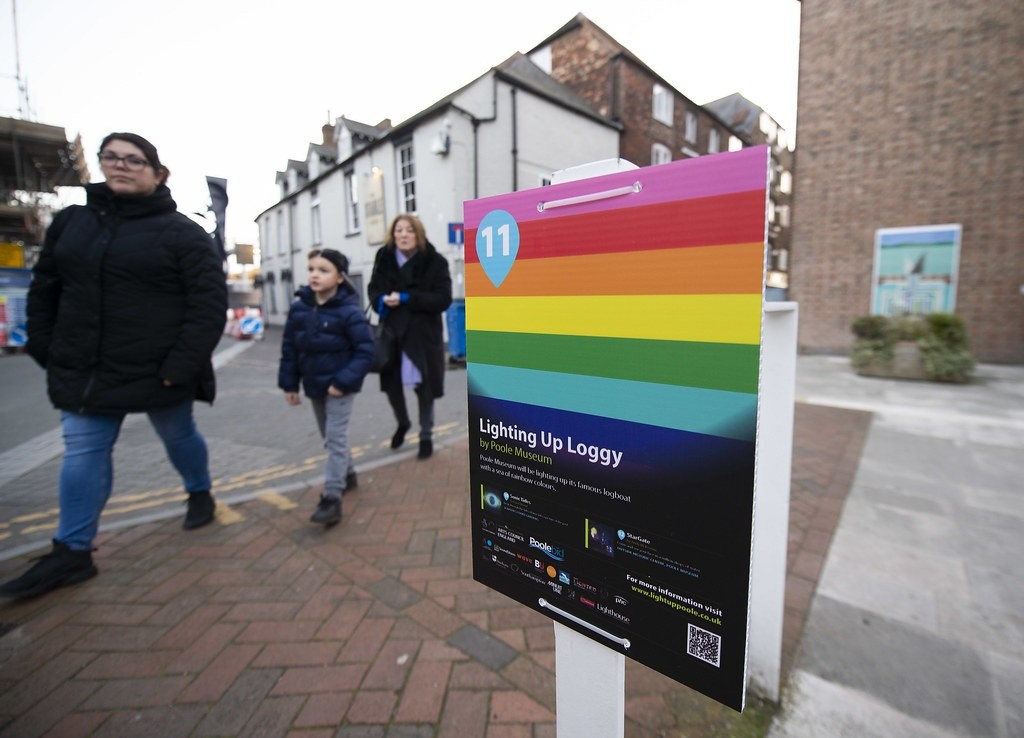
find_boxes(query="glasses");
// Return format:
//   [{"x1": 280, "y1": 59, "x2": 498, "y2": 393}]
[{"x1": 97, "y1": 151, "x2": 153, "y2": 173}]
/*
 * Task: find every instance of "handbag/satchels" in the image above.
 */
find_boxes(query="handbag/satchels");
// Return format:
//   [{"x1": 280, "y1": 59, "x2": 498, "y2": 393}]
[{"x1": 362, "y1": 293, "x2": 389, "y2": 373}]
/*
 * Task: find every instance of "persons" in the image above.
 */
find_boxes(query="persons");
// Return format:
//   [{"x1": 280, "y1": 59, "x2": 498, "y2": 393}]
[
  {"x1": 368, "y1": 214, "x2": 453, "y2": 458},
  {"x1": 279, "y1": 249, "x2": 377, "y2": 523},
  {"x1": 1, "y1": 133, "x2": 228, "y2": 597}
]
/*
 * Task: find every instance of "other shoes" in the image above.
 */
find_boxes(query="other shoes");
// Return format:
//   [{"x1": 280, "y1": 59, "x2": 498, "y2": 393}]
[
  {"x1": 183, "y1": 491, "x2": 215, "y2": 528},
  {"x1": 343, "y1": 469, "x2": 357, "y2": 493},
  {"x1": 418, "y1": 439, "x2": 432, "y2": 459},
  {"x1": 392, "y1": 422, "x2": 410, "y2": 449},
  {"x1": 311, "y1": 495, "x2": 341, "y2": 521},
  {"x1": 1, "y1": 538, "x2": 98, "y2": 598}
]
